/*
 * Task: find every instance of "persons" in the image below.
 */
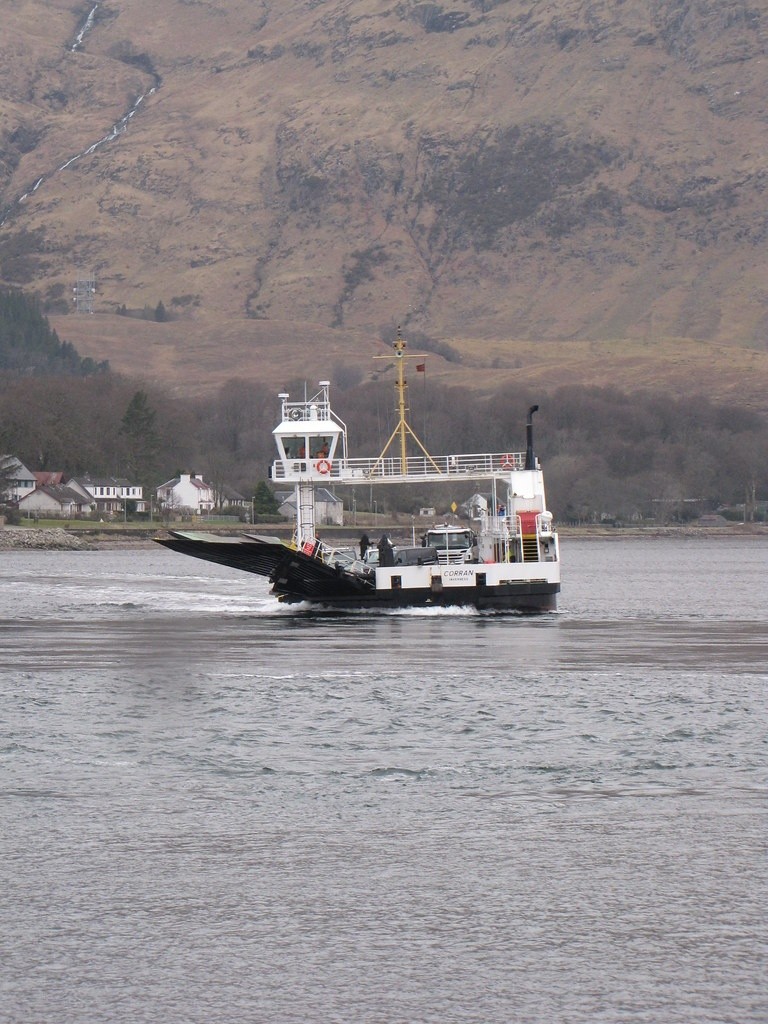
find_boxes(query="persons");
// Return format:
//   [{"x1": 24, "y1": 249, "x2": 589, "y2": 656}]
[
  {"x1": 285, "y1": 441, "x2": 331, "y2": 472},
  {"x1": 313, "y1": 531, "x2": 324, "y2": 561},
  {"x1": 497, "y1": 504, "x2": 506, "y2": 517}
]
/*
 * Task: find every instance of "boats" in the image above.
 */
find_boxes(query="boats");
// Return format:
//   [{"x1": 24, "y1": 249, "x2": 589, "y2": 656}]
[{"x1": 147, "y1": 326, "x2": 562, "y2": 615}]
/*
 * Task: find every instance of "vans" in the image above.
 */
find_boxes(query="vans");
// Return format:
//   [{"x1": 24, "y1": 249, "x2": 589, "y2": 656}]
[{"x1": 366, "y1": 547, "x2": 439, "y2": 569}]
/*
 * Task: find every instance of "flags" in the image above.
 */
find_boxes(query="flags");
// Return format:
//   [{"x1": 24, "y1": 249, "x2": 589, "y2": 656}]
[{"x1": 416, "y1": 364, "x2": 425, "y2": 372}]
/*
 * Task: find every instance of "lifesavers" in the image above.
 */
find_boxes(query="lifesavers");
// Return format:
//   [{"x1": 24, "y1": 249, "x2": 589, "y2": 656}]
[
  {"x1": 316, "y1": 460, "x2": 331, "y2": 474},
  {"x1": 500, "y1": 455, "x2": 516, "y2": 469}
]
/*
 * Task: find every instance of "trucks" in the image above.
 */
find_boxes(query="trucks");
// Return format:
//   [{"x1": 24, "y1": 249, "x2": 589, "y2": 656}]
[{"x1": 420, "y1": 524, "x2": 478, "y2": 566}]
